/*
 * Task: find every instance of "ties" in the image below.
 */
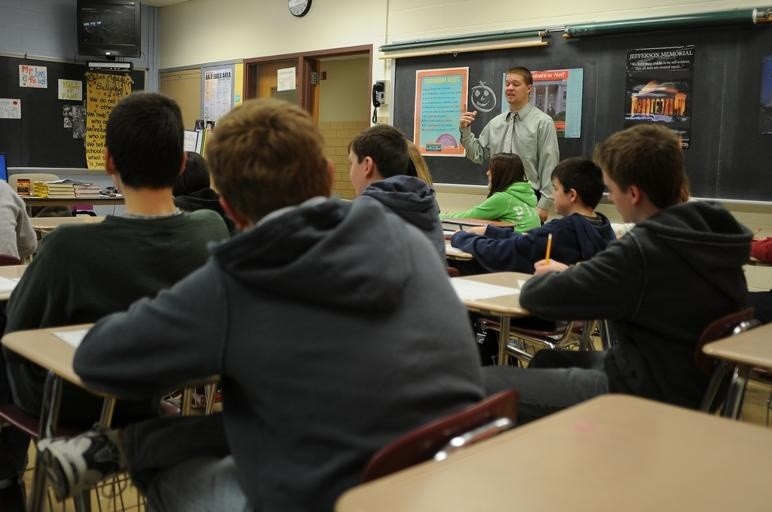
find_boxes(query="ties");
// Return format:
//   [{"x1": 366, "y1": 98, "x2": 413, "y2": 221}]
[{"x1": 502, "y1": 113, "x2": 518, "y2": 154}]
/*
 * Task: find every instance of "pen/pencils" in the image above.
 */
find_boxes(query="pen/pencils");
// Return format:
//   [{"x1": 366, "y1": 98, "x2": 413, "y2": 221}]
[
  {"x1": 545, "y1": 233, "x2": 552, "y2": 265},
  {"x1": 459, "y1": 224, "x2": 462, "y2": 231}
]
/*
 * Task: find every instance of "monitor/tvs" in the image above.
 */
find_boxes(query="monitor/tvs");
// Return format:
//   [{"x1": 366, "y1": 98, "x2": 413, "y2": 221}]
[{"x1": 77, "y1": 0, "x2": 141, "y2": 63}]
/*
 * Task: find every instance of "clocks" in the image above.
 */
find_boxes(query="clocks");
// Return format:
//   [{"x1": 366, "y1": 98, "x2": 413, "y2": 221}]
[{"x1": 287, "y1": 0, "x2": 312, "y2": 16}]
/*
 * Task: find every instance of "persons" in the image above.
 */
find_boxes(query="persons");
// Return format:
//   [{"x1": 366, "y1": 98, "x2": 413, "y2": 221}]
[
  {"x1": 459, "y1": 67, "x2": 560, "y2": 231},
  {"x1": 172, "y1": 152, "x2": 237, "y2": 239},
  {"x1": 482, "y1": 124, "x2": 754, "y2": 426},
  {"x1": 0, "y1": 92, "x2": 236, "y2": 511},
  {"x1": 440, "y1": 154, "x2": 618, "y2": 276},
  {"x1": 746, "y1": 226, "x2": 771, "y2": 268},
  {"x1": 436, "y1": 150, "x2": 541, "y2": 236},
  {"x1": 30, "y1": 96, "x2": 487, "y2": 511},
  {"x1": 0, "y1": 177, "x2": 38, "y2": 269},
  {"x1": 348, "y1": 124, "x2": 449, "y2": 275}
]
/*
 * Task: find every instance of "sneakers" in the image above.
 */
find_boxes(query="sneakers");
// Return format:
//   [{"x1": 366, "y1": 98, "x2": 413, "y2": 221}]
[{"x1": 34, "y1": 431, "x2": 123, "y2": 504}]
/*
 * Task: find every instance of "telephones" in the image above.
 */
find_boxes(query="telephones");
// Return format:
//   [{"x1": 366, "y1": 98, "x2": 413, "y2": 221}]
[{"x1": 373, "y1": 80, "x2": 390, "y2": 107}]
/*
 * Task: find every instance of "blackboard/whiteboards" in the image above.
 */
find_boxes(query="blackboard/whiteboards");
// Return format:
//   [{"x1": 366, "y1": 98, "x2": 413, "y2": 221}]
[
  {"x1": 390, "y1": 7, "x2": 772, "y2": 206},
  {"x1": 0, "y1": 51, "x2": 149, "y2": 170}
]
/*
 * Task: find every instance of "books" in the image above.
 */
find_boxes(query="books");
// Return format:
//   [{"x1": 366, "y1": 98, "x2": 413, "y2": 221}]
[{"x1": 16, "y1": 178, "x2": 102, "y2": 199}]
